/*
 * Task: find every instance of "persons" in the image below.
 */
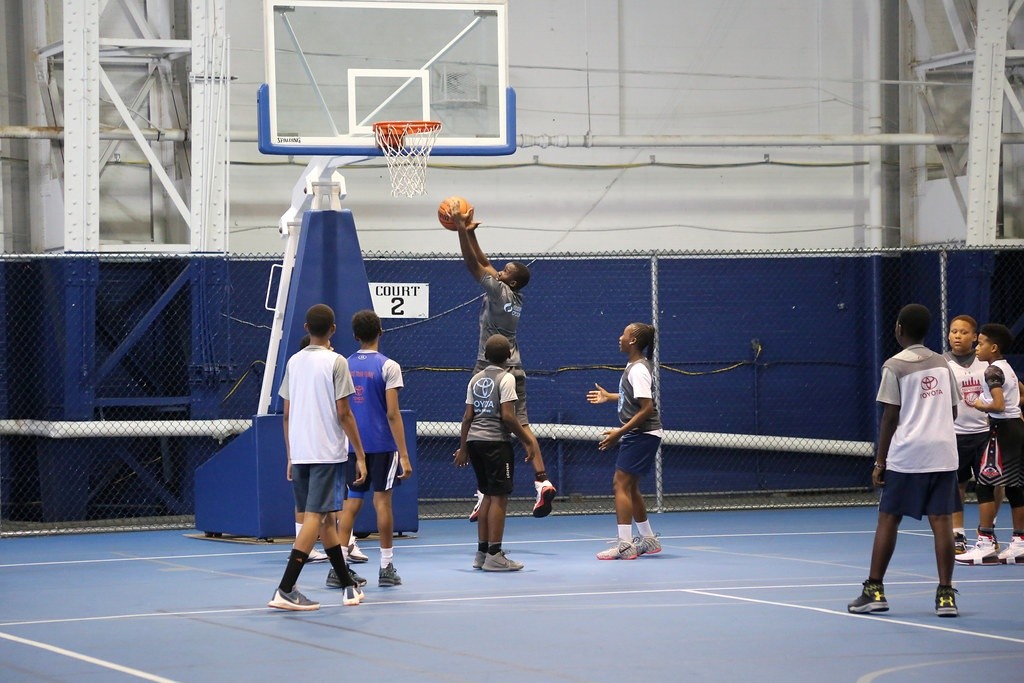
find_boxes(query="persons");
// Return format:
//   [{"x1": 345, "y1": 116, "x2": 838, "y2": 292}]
[
  {"x1": 269, "y1": 303, "x2": 366, "y2": 610},
  {"x1": 287, "y1": 309, "x2": 412, "y2": 588},
  {"x1": 847, "y1": 304, "x2": 964, "y2": 617},
  {"x1": 586, "y1": 323, "x2": 663, "y2": 561},
  {"x1": 453, "y1": 335, "x2": 538, "y2": 573},
  {"x1": 444, "y1": 200, "x2": 556, "y2": 523},
  {"x1": 941, "y1": 313, "x2": 1024, "y2": 567}
]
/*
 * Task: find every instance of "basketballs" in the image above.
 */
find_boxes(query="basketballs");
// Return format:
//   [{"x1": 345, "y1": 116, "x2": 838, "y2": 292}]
[{"x1": 438, "y1": 197, "x2": 473, "y2": 231}]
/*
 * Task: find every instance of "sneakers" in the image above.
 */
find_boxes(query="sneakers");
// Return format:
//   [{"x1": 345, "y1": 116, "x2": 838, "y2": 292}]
[
  {"x1": 308, "y1": 548, "x2": 329, "y2": 561},
  {"x1": 473, "y1": 550, "x2": 488, "y2": 568},
  {"x1": 469, "y1": 491, "x2": 486, "y2": 522},
  {"x1": 953, "y1": 539, "x2": 1000, "y2": 565},
  {"x1": 269, "y1": 588, "x2": 320, "y2": 609},
  {"x1": 848, "y1": 579, "x2": 889, "y2": 613},
  {"x1": 935, "y1": 586, "x2": 958, "y2": 616},
  {"x1": 327, "y1": 567, "x2": 366, "y2": 587},
  {"x1": 532, "y1": 480, "x2": 556, "y2": 517},
  {"x1": 631, "y1": 529, "x2": 661, "y2": 555},
  {"x1": 379, "y1": 564, "x2": 401, "y2": 587},
  {"x1": 343, "y1": 587, "x2": 364, "y2": 605},
  {"x1": 596, "y1": 539, "x2": 637, "y2": 559},
  {"x1": 976, "y1": 528, "x2": 1000, "y2": 551},
  {"x1": 482, "y1": 553, "x2": 524, "y2": 572},
  {"x1": 996, "y1": 537, "x2": 1024, "y2": 565},
  {"x1": 952, "y1": 531, "x2": 966, "y2": 554},
  {"x1": 348, "y1": 536, "x2": 368, "y2": 562}
]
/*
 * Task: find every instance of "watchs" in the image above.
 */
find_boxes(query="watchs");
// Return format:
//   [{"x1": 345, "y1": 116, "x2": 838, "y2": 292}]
[{"x1": 873, "y1": 461, "x2": 886, "y2": 469}]
